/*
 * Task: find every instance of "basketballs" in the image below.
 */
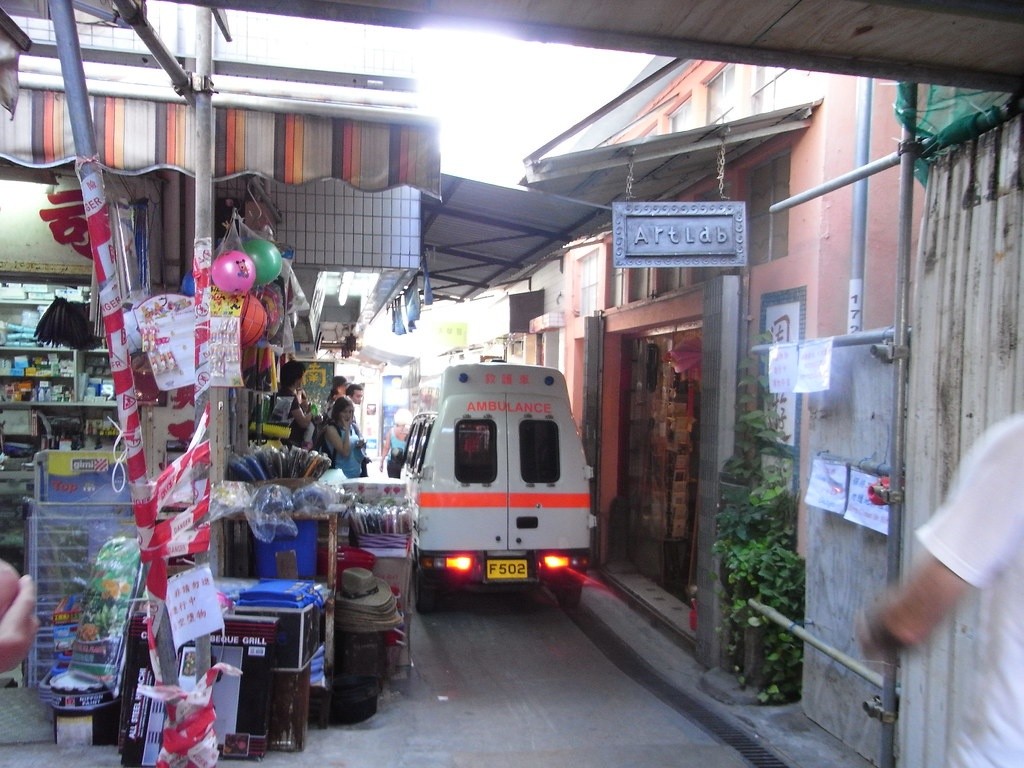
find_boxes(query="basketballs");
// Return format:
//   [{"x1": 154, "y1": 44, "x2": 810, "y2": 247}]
[{"x1": 241, "y1": 293, "x2": 267, "y2": 350}]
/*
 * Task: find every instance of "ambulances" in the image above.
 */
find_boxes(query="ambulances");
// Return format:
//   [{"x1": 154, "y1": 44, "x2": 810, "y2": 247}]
[{"x1": 399, "y1": 364, "x2": 598, "y2": 615}]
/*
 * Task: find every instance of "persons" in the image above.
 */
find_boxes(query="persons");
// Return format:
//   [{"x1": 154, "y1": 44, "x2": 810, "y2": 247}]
[
  {"x1": 855, "y1": 412, "x2": 1024, "y2": 768},
  {"x1": 379, "y1": 408, "x2": 414, "y2": 480},
  {"x1": 268, "y1": 358, "x2": 365, "y2": 478},
  {"x1": 0, "y1": 558, "x2": 41, "y2": 676}
]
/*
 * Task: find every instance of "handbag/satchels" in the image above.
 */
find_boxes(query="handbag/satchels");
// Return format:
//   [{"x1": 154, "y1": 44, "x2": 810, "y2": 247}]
[{"x1": 352, "y1": 425, "x2": 372, "y2": 477}]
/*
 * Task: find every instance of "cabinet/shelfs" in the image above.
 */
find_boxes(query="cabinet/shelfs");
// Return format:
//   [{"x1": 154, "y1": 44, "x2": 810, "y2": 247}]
[{"x1": 0, "y1": 283, "x2": 126, "y2": 548}]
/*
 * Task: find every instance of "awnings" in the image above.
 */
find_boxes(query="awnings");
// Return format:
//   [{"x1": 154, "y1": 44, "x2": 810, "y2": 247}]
[
  {"x1": 386, "y1": 88, "x2": 832, "y2": 320},
  {"x1": 0, "y1": 62, "x2": 457, "y2": 203}
]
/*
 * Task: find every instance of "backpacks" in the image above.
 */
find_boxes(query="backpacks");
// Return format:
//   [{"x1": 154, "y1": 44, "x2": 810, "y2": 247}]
[{"x1": 313, "y1": 423, "x2": 342, "y2": 469}]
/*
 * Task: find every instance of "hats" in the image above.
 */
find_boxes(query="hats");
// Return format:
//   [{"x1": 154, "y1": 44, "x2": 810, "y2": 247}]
[{"x1": 334, "y1": 567, "x2": 403, "y2": 632}]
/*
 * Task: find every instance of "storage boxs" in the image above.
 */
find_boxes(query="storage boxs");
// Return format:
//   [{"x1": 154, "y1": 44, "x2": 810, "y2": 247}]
[
  {"x1": 341, "y1": 476, "x2": 408, "y2": 500},
  {"x1": 395, "y1": 606, "x2": 414, "y2": 667},
  {"x1": 373, "y1": 556, "x2": 414, "y2": 611},
  {"x1": 119, "y1": 594, "x2": 324, "y2": 768}
]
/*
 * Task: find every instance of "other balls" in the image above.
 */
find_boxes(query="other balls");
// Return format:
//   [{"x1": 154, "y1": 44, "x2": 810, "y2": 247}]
[
  {"x1": 242, "y1": 240, "x2": 283, "y2": 286},
  {"x1": 211, "y1": 251, "x2": 255, "y2": 293}
]
